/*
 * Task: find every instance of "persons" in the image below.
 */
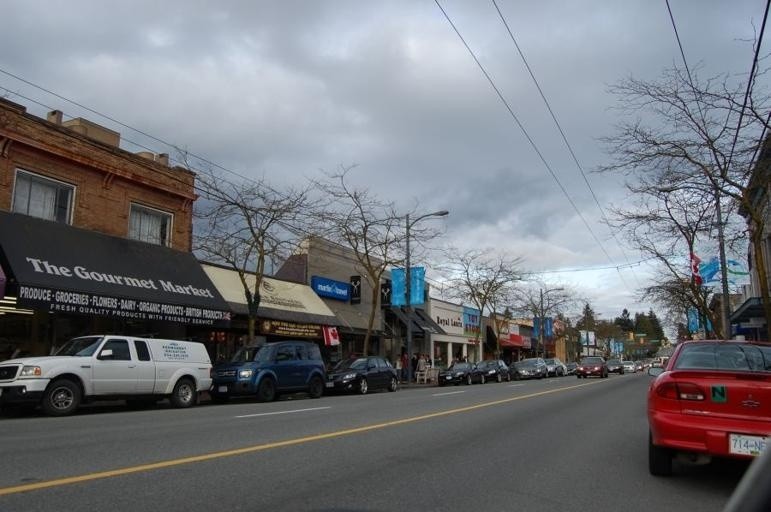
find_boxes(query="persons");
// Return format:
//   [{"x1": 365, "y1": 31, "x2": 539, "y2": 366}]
[{"x1": 401, "y1": 351, "x2": 466, "y2": 381}]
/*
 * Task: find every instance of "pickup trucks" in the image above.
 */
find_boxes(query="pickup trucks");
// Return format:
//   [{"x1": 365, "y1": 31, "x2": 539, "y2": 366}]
[{"x1": 0, "y1": 334, "x2": 212, "y2": 415}]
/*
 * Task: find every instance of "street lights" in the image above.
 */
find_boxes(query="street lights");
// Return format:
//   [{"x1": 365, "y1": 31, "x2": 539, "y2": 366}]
[
  {"x1": 406, "y1": 209, "x2": 450, "y2": 387},
  {"x1": 585, "y1": 313, "x2": 603, "y2": 356},
  {"x1": 540, "y1": 287, "x2": 564, "y2": 357}
]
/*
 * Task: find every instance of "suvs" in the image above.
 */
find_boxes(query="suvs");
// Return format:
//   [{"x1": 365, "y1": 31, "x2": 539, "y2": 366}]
[{"x1": 210, "y1": 341, "x2": 328, "y2": 402}]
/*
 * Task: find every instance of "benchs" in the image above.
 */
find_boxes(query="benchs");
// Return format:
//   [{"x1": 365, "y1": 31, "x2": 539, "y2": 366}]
[{"x1": 416, "y1": 367, "x2": 440, "y2": 384}]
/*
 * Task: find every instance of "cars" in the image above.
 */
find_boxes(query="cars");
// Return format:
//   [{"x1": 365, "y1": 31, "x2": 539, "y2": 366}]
[
  {"x1": 608, "y1": 360, "x2": 624, "y2": 375},
  {"x1": 647, "y1": 340, "x2": 771, "y2": 476},
  {"x1": 644, "y1": 356, "x2": 670, "y2": 368},
  {"x1": 635, "y1": 361, "x2": 644, "y2": 372},
  {"x1": 623, "y1": 361, "x2": 636, "y2": 372},
  {"x1": 508, "y1": 362, "x2": 519, "y2": 381},
  {"x1": 577, "y1": 357, "x2": 609, "y2": 379},
  {"x1": 566, "y1": 363, "x2": 579, "y2": 375},
  {"x1": 325, "y1": 356, "x2": 400, "y2": 394},
  {"x1": 438, "y1": 362, "x2": 486, "y2": 386},
  {"x1": 518, "y1": 358, "x2": 547, "y2": 380},
  {"x1": 476, "y1": 359, "x2": 511, "y2": 382},
  {"x1": 544, "y1": 358, "x2": 567, "y2": 376}
]
